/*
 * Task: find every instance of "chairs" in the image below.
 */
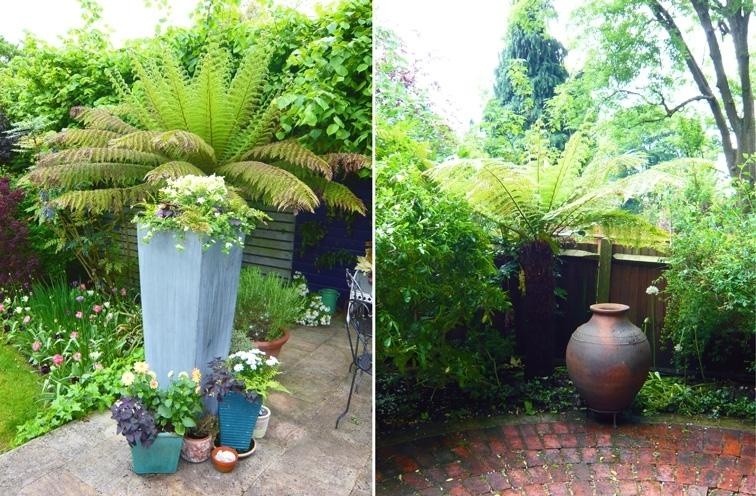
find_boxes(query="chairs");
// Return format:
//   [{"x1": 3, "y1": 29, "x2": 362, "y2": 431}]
[{"x1": 335, "y1": 298, "x2": 371, "y2": 431}]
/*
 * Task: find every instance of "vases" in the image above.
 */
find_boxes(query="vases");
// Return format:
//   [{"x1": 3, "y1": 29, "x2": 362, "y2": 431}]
[
  {"x1": 181, "y1": 432, "x2": 212, "y2": 464},
  {"x1": 129, "y1": 432, "x2": 183, "y2": 475},
  {"x1": 211, "y1": 447, "x2": 238, "y2": 473},
  {"x1": 218, "y1": 391, "x2": 262, "y2": 451},
  {"x1": 565, "y1": 303, "x2": 652, "y2": 421}
]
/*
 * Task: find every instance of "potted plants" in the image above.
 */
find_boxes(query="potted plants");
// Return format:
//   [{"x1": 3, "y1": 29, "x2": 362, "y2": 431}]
[{"x1": 235, "y1": 263, "x2": 302, "y2": 361}]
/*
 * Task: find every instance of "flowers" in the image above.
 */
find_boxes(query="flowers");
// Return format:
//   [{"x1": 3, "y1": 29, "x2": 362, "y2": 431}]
[
  {"x1": 113, "y1": 349, "x2": 290, "y2": 449},
  {"x1": 288, "y1": 271, "x2": 332, "y2": 327},
  {"x1": 129, "y1": 173, "x2": 275, "y2": 255}
]
